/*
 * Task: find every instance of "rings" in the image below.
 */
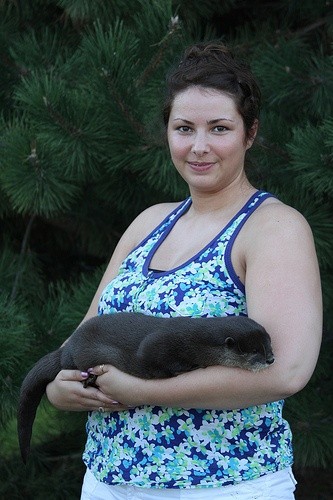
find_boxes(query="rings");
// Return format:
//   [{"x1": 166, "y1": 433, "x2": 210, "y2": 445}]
[{"x1": 99, "y1": 363, "x2": 105, "y2": 373}]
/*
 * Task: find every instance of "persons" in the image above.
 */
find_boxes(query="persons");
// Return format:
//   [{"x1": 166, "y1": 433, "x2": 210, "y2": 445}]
[{"x1": 44, "y1": 44, "x2": 326, "y2": 500}]
